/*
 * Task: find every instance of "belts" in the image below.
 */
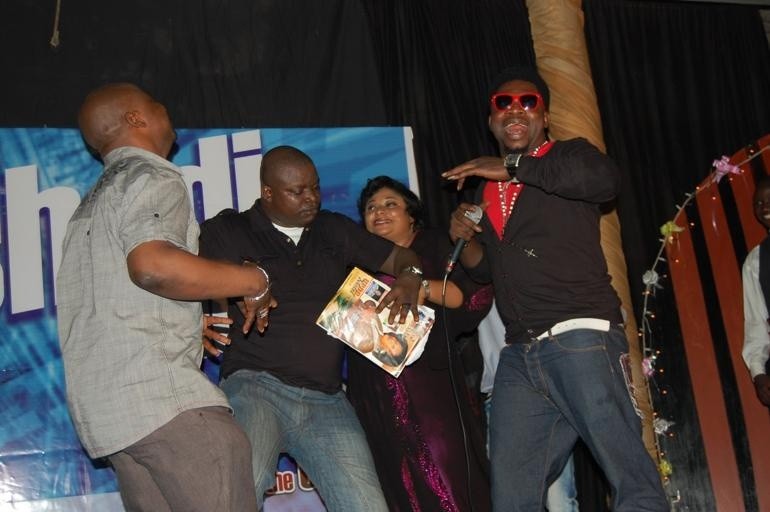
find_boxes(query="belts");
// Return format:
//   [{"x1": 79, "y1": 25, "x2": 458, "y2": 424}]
[{"x1": 536, "y1": 318, "x2": 610, "y2": 341}]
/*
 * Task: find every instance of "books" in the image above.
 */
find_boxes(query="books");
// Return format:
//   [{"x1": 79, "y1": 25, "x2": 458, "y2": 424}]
[{"x1": 314, "y1": 266, "x2": 435, "y2": 380}]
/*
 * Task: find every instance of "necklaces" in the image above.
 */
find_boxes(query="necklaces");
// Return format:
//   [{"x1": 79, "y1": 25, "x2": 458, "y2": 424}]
[{"x1": 497, "y1": 140, "x2": 549, "y2": 235}]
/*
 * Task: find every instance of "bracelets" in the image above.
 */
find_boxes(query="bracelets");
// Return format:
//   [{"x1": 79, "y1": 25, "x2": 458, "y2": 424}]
[
  {"x1": 245, "y1": 260, "x2": 269, "y2": 302},
  {"x1": 422, "y1": 280, "x2": 429, "y2": 300}
]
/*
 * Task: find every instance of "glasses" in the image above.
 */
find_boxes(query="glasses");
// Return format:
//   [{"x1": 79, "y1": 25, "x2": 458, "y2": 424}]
[{"x1": 490, "y1": 93, "x2": 546, "y2": 113}]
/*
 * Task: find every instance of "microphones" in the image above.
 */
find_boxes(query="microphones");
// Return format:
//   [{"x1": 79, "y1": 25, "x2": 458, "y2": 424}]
[{"x1": 444, "y1": 204, "x2": 485, "y2": 273}]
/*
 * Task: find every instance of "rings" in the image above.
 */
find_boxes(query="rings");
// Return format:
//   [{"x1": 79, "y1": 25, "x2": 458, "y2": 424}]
[
  {"x1": 402, "y1": 303, "x2": 411, "y2": 309},
  {"x1": 255, "y1": 307, "x2": 269, "y2": 318}
]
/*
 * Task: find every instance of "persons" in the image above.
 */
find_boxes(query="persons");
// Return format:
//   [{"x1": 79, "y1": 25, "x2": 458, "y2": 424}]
[
  {"x1": 438, "y1": 69, "x2": 669, "y2": 511},
  {"x1": 741, "y1": 177, "x2": 770, "y2": 414},
  {"x1": 197, "y1": 145, "x2": 426, "y2": 512},
  {"x1": 476, "y1": 299, "x2": 580, "y2": 512},
  {"x1": 344, "y1": 175, "x2": 494, "y2": 512},
  {"x1": 54, "y1": 79, "x2": 280, "y2": 512}
]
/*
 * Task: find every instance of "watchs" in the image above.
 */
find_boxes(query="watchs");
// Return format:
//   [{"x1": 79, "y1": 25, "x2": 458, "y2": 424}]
[
  {"x1": 503, "y1": 152, "x2": 523, "y2": 177},
  {"x1": 400, "y1": 264, "x2": 424, "y2": 280}
]
